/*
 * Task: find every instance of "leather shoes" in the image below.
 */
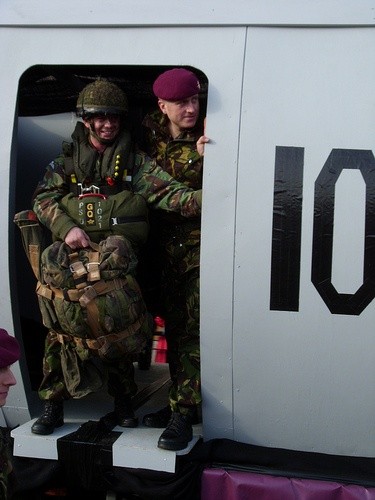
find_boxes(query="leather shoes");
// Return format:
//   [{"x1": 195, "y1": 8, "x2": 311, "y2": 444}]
[
  {"x1": 114, "y1": 395, "x2": 139, "y2": 429},
  {"x1": 143, "y1": 403, "x2": 174, "y2": 427},
  {"x1": 158, "y1": 423, "x2": 193, "y2": 451},
  {"x1": 31, "y1": 396, "x2": 64, "y2": 436}
]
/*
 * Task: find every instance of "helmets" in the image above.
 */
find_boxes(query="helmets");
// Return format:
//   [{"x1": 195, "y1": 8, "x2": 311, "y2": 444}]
[{"x1": 76, "y1": 80, "x2": 130, "y2": 117}]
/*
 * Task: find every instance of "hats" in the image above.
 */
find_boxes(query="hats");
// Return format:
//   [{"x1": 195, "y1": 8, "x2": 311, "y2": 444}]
[
  {"x1": 0, "y1": 327, "x2": 21, "y2": 371},
  {"x1": 153, "y1": 68, "x2": 201, "y2": 102}
]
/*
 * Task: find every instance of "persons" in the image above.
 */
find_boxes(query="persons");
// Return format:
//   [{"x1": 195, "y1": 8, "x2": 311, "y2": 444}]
[
  {"x1": 31, "y1": 68, "x2": 208, "y2": 451},
  {"x1": 0, "y1": 327, "x2": 21, "y2": 500}
]
